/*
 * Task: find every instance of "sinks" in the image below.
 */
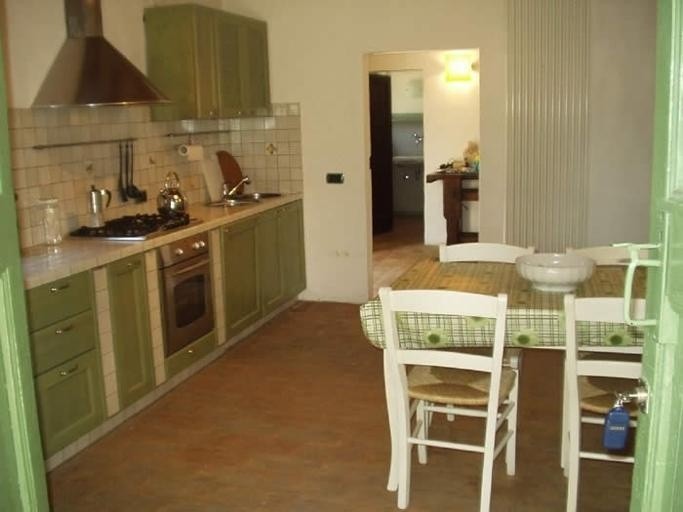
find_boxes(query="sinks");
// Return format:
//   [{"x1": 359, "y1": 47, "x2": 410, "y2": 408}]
[
  {"x1": 206, "y1": 200, "x2": 252, "y2": 208},
  {"x1": 242, "y1": 192, "x2": 280, "y2": 199}
]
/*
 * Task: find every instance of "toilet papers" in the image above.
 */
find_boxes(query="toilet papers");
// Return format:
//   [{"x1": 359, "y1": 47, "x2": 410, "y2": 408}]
[{"x1": 178, "y1": 144, "x2": 204, "y2": 162}]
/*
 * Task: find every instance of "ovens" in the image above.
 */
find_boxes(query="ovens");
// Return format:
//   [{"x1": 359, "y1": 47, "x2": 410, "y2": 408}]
[{"x1": 155, "y1": 254, "x2": 223, "y2": 381}]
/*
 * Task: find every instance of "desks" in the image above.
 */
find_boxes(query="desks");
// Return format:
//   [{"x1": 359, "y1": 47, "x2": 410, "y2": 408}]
[
  {"x1": 427, "y1": 171, "x2": 479, "y2": 246},
  {"x1": 359, "y1": 259, "x2": 649, "y2": 493}
]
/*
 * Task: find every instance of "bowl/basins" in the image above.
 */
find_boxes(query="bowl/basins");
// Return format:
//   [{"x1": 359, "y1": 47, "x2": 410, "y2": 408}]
[{"x1": 515, "y1": 252, "x2": 596, "y2": 294}]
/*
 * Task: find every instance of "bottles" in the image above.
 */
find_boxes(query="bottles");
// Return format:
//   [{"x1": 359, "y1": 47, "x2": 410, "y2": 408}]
[{"x1": 38, "y1": 199, "x2": 62, "y2": 248}]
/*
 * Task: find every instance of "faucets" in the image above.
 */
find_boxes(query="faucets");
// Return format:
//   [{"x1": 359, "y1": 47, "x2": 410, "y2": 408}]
[
  {"x1": 412, "y1": 132, "x2": 423, "y2": 146},
  {"x1": 228, "y1": 176, "x2": 252, "y2": 199}
]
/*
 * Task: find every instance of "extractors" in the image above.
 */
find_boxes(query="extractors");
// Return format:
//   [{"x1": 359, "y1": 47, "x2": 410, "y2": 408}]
[{"x1": 34, "y1": 1, "x2": 171, "y2": 106}]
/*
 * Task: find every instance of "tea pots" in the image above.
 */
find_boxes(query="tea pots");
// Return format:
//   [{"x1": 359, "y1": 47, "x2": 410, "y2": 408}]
[
  {"x1": 155, "y1": 170, "x2": 187, "y2": 216},
  {"x1": 84, "y1": 184, "x2": 111, "y2": 229}
]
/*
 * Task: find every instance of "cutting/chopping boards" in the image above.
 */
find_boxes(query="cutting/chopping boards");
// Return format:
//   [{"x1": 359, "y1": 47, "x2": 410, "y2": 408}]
[
  {"x1": 198, "y1": 160, "x2": 226, "y2": 203},
  {"x1": 216, "y1": 150, "x2": 243, "y2": 196}
]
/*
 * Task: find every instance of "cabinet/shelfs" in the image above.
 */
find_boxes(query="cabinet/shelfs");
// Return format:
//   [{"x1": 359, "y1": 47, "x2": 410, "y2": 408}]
[
  {"x1": 220, "y1": 215, "x2": 264, "y2": 341},
  {"x1": 214, "y1": 8, "x2": 273, "y2": 119},
  {"x1": 26, "y1": 268, "x2": 109, "y2": 462},
  {"x1": 143, "y1": 3, "x2": 218, "y2": 122},
  {"x1": 105, "y1": 252, "x2": 157, "y2": 412},
  {"x1": 256, "y1": 199, "x2": 307, "y2": 317}
]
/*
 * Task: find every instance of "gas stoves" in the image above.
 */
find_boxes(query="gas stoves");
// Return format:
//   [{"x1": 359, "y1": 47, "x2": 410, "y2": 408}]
[{"x1": 66, "y1": 214, "x2": 208, "y2": 270}]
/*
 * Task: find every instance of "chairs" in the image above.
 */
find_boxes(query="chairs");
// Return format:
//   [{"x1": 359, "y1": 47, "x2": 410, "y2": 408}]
[
  {"x1": 379, "y1": 286, "x2": 520, "y2": 512},
  {"x1": 559, "y1": 243, "x2": 650, "y2": 469},
  {"x1": 560, "y1": 293, "x2": 644, "y2": 512},
  {"x1": 425, "y1": 241, "x2": 534, "y2": 440}
]
